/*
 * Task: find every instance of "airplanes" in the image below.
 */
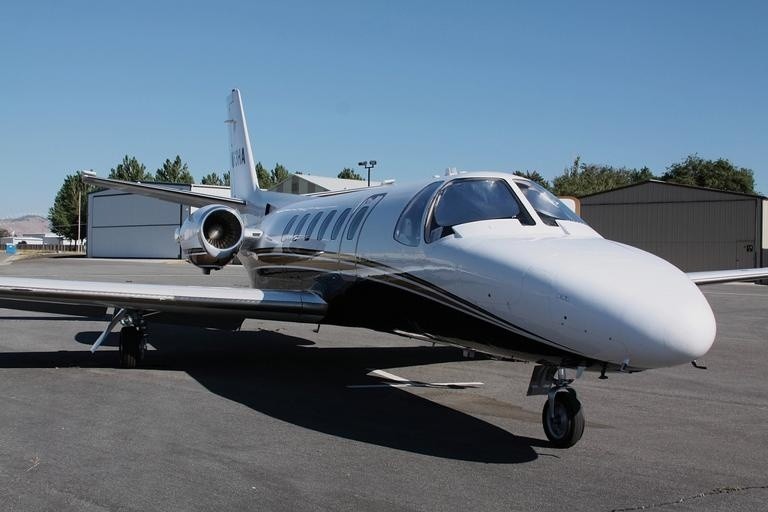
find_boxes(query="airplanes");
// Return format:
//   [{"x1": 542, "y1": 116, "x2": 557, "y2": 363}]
[{"x1": 0, "y1": 88, "x2": 768, "y2": 449}]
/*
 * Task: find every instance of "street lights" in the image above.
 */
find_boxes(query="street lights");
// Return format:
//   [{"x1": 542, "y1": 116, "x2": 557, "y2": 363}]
[{"x1": 358, "y1": 160, "x2": 377, "y2": 187}]
